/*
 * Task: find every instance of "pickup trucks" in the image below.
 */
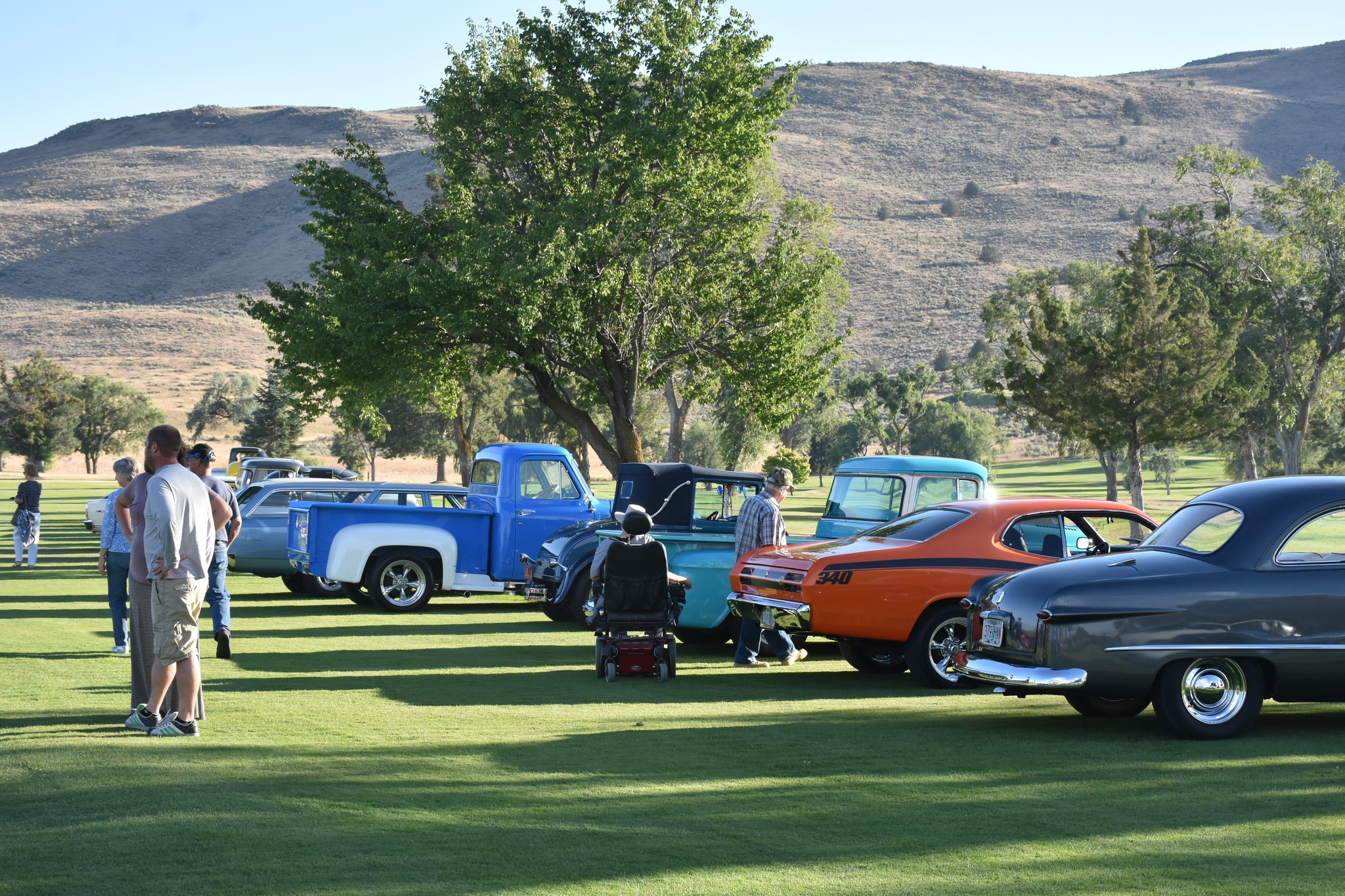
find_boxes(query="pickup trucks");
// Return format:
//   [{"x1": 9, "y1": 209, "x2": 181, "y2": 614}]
[
  {"x1": 82, "y1": 445, "x2": 420, "y2": 599},
  {"x1": 283, "y1": 440, "x2": 705, "y2": 614},
  {"x1": 581, "y1": 454, "x2": 990, "y2": 655}
]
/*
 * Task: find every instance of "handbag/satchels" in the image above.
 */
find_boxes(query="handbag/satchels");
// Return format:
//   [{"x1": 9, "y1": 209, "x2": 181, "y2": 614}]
[
  {"x1": 10, "y1": 508, "x2": 20, "y2": 526},
  {"x1": 583, "y1": 588, "x2": 604, "y2": 613}
]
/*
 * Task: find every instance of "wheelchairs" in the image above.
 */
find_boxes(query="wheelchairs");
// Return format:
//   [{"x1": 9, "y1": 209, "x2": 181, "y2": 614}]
[{"x1": 593, "y1": 580, "x2": 686, "y2": 682}]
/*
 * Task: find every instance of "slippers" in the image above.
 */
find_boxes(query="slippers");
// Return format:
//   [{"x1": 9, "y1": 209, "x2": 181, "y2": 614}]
[{"x1": 12, "y1": 564, "x2": 20, "y2": 567}]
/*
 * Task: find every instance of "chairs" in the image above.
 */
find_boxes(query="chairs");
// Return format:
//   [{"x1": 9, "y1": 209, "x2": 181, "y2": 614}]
[
  {"x1": 1042, "y1": 534, "x2": 1071, "y2": 558},
  {"x1": 1005, "y1": 527, "x2": 1028, "y2": 552}
]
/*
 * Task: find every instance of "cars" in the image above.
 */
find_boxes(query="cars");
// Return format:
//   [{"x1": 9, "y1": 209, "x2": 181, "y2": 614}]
[
  {"x1": 726, "y1": 495, "x2": 1189, "y2": 691},
  {"x1": 940, "y1": 474, "x2": 1345, "y2": 742}
]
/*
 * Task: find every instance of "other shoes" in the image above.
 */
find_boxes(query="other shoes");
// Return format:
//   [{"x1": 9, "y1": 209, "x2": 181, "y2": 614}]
[
  {"x1": 216, "y1": 628, "x2": 231, "y2": 659},
  {"x1": 112, "y1": 645, "x2": 130, "y2": 654}
]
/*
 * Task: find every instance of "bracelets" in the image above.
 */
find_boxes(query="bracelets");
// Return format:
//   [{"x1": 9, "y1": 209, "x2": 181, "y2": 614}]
[{"x1": 99, "y1": 551, "x2": 107, "y2": 557}]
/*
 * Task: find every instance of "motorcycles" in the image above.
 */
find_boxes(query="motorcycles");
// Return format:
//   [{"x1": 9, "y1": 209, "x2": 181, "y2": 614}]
[{"x1": 518, "y1": 462, "x2": 791, "y2": 635}]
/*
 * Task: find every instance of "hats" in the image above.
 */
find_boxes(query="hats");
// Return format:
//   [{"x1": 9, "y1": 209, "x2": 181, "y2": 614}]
[
  {"x1": 766, "y1": 467, "x2": 798, "y2": 489},
  {"x1": 187, "y1": 443, "x2": 216, "y2": 462},
  {"x1": 615, "y1": 504, "x2": 654, "y2": 526}
]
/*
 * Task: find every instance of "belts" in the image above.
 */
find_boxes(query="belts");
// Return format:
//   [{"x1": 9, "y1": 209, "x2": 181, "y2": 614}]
[{"x1": 215, "y1": 540, "x2": 226, "y2": 544}]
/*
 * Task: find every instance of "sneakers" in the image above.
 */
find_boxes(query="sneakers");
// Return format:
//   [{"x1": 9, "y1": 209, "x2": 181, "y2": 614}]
[
  {"x1": 147, "y1": 709, "x2": 200, "y2": 737},
  {"x1": 781, "y1": 649, "x2": 807, "y2": 666},
  {"x1": 125, "y1": 703, "x2": 162, "y2": 732},
  {"x1": 733, "y1": 660, "x2": 769, "y2": 668}
]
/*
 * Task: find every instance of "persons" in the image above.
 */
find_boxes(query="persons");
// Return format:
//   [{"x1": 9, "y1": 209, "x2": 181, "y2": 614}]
[
  {"x1": 732, "y1": 467, "x2": 807, "y2": 668},
  {"x1": 590, "y1": 504, "x2": 693, "y2": 590},
  {"x1": 1013, "y1": 618, "x2": 1022, "y2": 643},
  {"x1": 115, "y1": 440, "x2": 233, "y2": 723},
  {"x1": 125, "y1": 425, "x2": 215, "y2": 736},
  {"x1": 13, "y1": 462, "x2": 42, "y2": 568},
  {"x1": 98, "y1": 457, "x2": 140, "y2": 654},
  {"x1": 185, "y1": 443, "x2": 242, "y2": 659}
]
